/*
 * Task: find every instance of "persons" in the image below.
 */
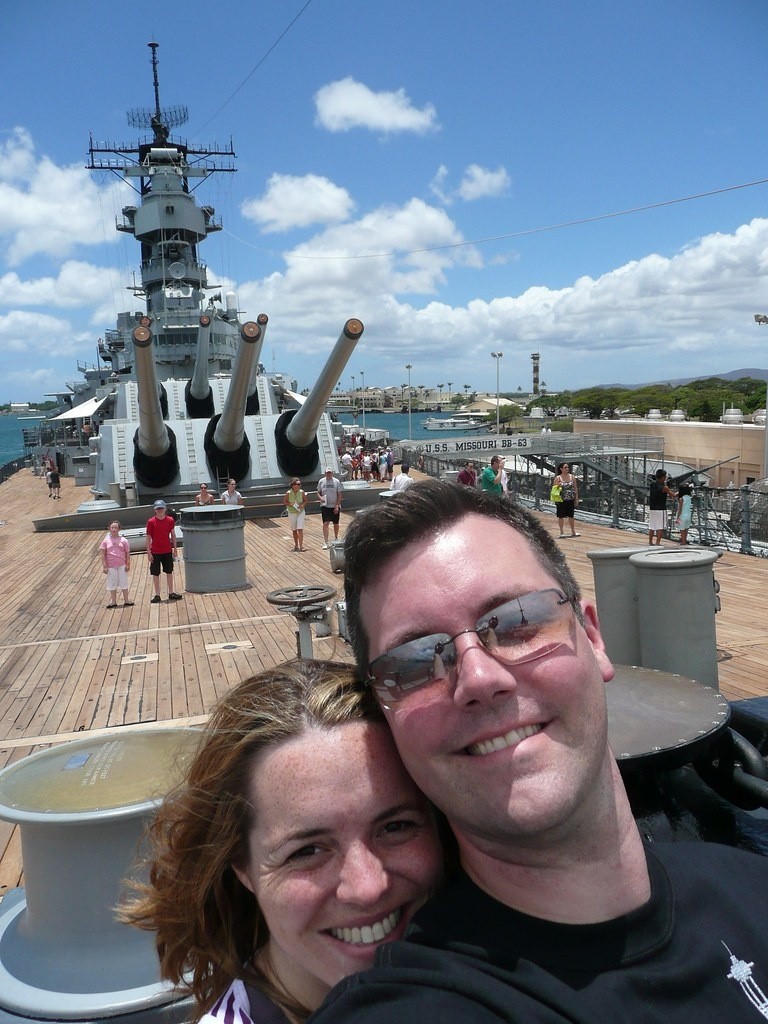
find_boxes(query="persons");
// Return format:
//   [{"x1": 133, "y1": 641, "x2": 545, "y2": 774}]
[
  {"x1": 317, "y1": 468, "x2": 342, "y2": 550},
  {"x1": 285, "y1": 478, "x2": 308, "y2": 551},
  {"x1": 100, "y1": 521, "x2": 135, "y2": 607},
  {"x1": 221, "y1": 479, "x2": 242, "y2": 505},
  {"x1": 145, "y1": 500, "x2": 183, "y2": 603},
  {"x1": 481, "y1": 456, "x2": 504, "y2": 496},
  {"x1": 676, "y1": 484, "x2": 691, "y2": 545},
  {"x1": 458, "y1": 462, "x2": 475, "y2": 486},
  {"x1": 390, "y1": 462, "x2": 413, "y2": 490},
  {"x1": 82, "y1": 421, "x2": 99, "y2": 446},
  {"x1": 300, "y1": 477, "x2": 767, "y2": 1023},
  {"x1": 540, "y1": 426, "x2": 551, "y2": 434},
  {"x1": 500, "y1": 457, "x2": 507, "y2": 492},
  {"x1": 649, "y1": 469, "x2": 679, "y2": 546},
  {"x1": 338, "y1": 434, "x2": 393, "y2": 483},
  {"x1": 39, "y1": 461, "x2": 61, "y2": 499},
  {"x1": 195, "y1": 483, "x2": 214, "y2": 506},
  {"x1": 118, "y1": 658, "x2": 447, "y2": 1024},
  {"x1": 554, "y1": 462, "x2": 581, "y2": 536},
  {"x1": 506, "y1": 428, "x2": 513, "y2": 435}
]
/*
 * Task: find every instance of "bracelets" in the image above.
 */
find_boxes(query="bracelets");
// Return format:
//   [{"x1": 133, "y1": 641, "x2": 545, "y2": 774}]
[
  {"x1": 291, "y1": 503, "x2": 293, "y2": 505},
  {"x1": 302, "y1": 505, "x2": 303, "y2": 507}
]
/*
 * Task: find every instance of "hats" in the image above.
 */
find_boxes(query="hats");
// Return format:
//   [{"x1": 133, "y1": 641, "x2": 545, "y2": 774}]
[
  {"x1": 402, "y1": 463, "x2": 409, "y2": 471},
  {"x1": 154, "y1": 499, "x2": 166, "y2": 509},
  {"x1": 325, "y1": 467, "x2": 332, "y2": 473}
]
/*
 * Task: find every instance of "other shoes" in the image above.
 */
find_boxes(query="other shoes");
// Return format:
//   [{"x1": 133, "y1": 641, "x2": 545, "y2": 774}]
[
  {"x1": 323, "y1": 543, "x2": 330, "y2": 550},
  {"x1": 106, "y1": 602, "x2": 117, "y2": 608},
  {"x1": 124, "y1": 601, "x2": 134, "y2": 605},
  {"x1": 559, "y1": 533, "x2": 569, "y2": 538},
  {"x1": 291, "y1": 547, "x2": 299, "y2": 551},
  {"x1": 573, "y1": 533, "x2": 581, "y2": 537},
  {"x1": 300, "y1": 548, "x2": 306, "y2": 552}
]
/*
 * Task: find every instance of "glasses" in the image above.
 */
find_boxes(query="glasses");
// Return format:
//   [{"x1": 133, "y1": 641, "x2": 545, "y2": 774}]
[
  {"x1": 200, "y1": 487, "x2": 206, "y2": 489},
  {"x1": 294, "y1": 484, "x2": 300, "y2": 486},
  {"x1": 365, "y1": 588, "x2": 580, "y2": 711}
]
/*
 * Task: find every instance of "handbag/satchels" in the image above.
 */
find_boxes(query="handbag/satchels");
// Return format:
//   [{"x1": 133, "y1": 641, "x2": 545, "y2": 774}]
[{"x1": 550, "y1": 485, "x2": 563, "y2": 502}]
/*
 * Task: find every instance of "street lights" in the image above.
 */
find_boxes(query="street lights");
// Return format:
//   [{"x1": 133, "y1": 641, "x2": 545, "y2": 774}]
[
  {"x1": 350, "y1": 376, "x2": 355, "y2": 406},
  {"x1": 490, "y1": 351, "x2": 503, "y2": 436},
  {"x1": 405, "y1": 364, "x2": 412, "y2": 440},
  {"x1": 359, "y1": 372, "x2": 366, "y2": 427}
]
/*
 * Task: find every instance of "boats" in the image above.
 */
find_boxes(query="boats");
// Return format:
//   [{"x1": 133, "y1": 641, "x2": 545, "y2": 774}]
[{"x1": 422, "y1": 411, "x2": 491, "y2": 431}]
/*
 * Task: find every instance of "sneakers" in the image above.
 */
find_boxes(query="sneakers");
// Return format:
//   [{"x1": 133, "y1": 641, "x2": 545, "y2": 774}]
[
  {"x1": 150, "y1": 596, "x2": 161, "y2": 603},
  {"x1": 169, "y1": 593, "x2": 182, "y2": 599}
]
